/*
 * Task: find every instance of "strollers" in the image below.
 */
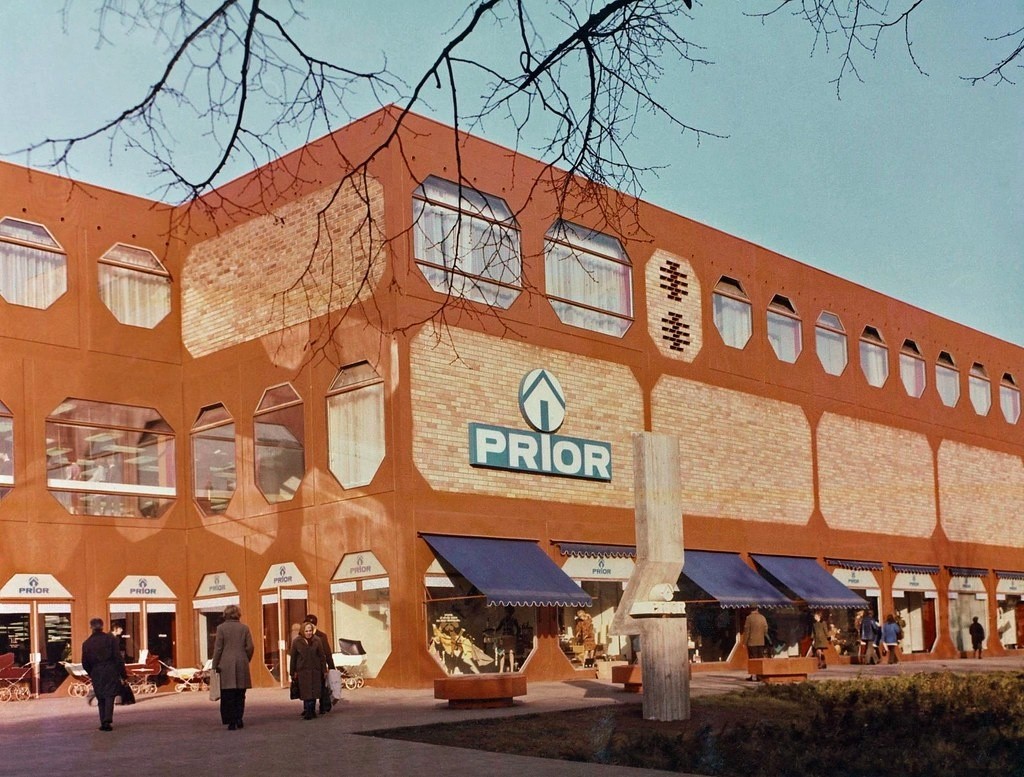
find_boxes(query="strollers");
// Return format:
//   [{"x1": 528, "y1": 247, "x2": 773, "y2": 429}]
[{"x1": 158, "y1": 660, "x2": 208, "y2": 693}]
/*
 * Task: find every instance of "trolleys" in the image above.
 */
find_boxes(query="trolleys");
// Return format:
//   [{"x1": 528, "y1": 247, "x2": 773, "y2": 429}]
[
  {"x1": 60, "y1": 655, "x2": 163, "y2": 707},
  {"x1": 331, "y1": 651, "x2": 369, "y2": 691},
  {"x1": 0, "y1": 652, "x2": 39, "y2": 705}
]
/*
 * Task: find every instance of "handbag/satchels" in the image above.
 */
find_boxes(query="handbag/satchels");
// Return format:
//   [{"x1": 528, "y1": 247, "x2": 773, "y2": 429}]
[
  {"x1": 208, "y1": 668, "x2": 222, "y2": 701},
  {"x1": 120, "y1": 683, "x2": 136, "y2": 705},
  {"x1": 290, "y1": 678, "x2": 300, "y2": 699},
  {"x1": 328, "y1": 667, "x2": 342, "y2": 700}
]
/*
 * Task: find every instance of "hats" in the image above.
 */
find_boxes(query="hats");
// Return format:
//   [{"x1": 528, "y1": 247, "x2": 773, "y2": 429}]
[
  {"x1": 574, "y1": 610, "x2": 592, "y2": 620},
  {"x1": 303, "y1": 615, "x2": 317, "y2": 626}
]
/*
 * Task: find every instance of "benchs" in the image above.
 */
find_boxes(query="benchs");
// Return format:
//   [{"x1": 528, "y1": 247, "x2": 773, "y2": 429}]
[
  {"x1": 611, "y1": 665, "x2": 693, "y2": 693},
  {"x1": 747, "y1": 658, "x2": 820, "y2": 685},
  {"x1": 433, "y1": 676, "x2": 527, "y2": 708}
]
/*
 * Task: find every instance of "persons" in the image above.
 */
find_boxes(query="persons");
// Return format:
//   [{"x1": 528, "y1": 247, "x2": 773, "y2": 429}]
[
  {"x1": 883, "y1": 614, "x2": 900, "y2": 665},
  {"x1": 969, "y1": 617, "x2": 985, "y2": 659},
  {"x1": 212, "y1": 606, "x2": 254, "y2": 730},
  {"x1": 574, "y1": 611, "x2": 596, "y2": 670},
  {"x1": 82, "y1": 617, "x2": 129, "y2": 732},
  {"x1": 742, "y1": 605, "x2": 771, "y2": 683},
  {"x1": 289, "y1": 614, "x2": 337, "y2": 721},
  {"x1": 811, "y1": 608, "x2": 831, "y2": 669},
  {"x1": 494, "y1": 606, "x2": 521, "y2": 674},
  {"x1": 858, "y1": 609, "x2": 880, "y2": 664}
]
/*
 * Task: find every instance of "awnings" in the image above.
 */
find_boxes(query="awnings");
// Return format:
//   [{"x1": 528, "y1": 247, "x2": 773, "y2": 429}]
[
  {"x1": 678, "y1": 549, "x2": 793, "y2": 610},
  {"x1": 416, "y1": 533, "x2": 592, "y2": 609},
  {"x1": 551, "y1": 539, "x2": 637, "y2": 558},
  {"x1": 887, "y1": 561, "x2": 940, "y2": 575},
  {"x1": 748, "y1": 553, "x2": 871, "y2": 610},
  {"x1": 993, "y1": 569, "x2": 1024, "y2": 580},
  {"x1": 823, "y1": 556, "x2": 884, "y2": 572},
  {"x1": 944, "y1": 565, "x2": 989, "y2": 577}
]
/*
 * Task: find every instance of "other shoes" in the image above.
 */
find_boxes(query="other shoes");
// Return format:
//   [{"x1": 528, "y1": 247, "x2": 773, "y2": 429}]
[
  {"x1": 228, "y1": 725, "x2": 235, "y2": 729},
  {"x1": 304, "y1": 711, "x2": 316, "y2": 720},
  {"x1": 236, "y1": 719, "x2": 243, "y2": 728},
  {"x1": 319, "y1": 711, "x2": 325, "y2": 714},
  {"x1": 99, "y1": 726, "x2": 105, "y2": 730},
  {"x1": 105, "y1": 726, "x2": 112, "y2": 731}
]
